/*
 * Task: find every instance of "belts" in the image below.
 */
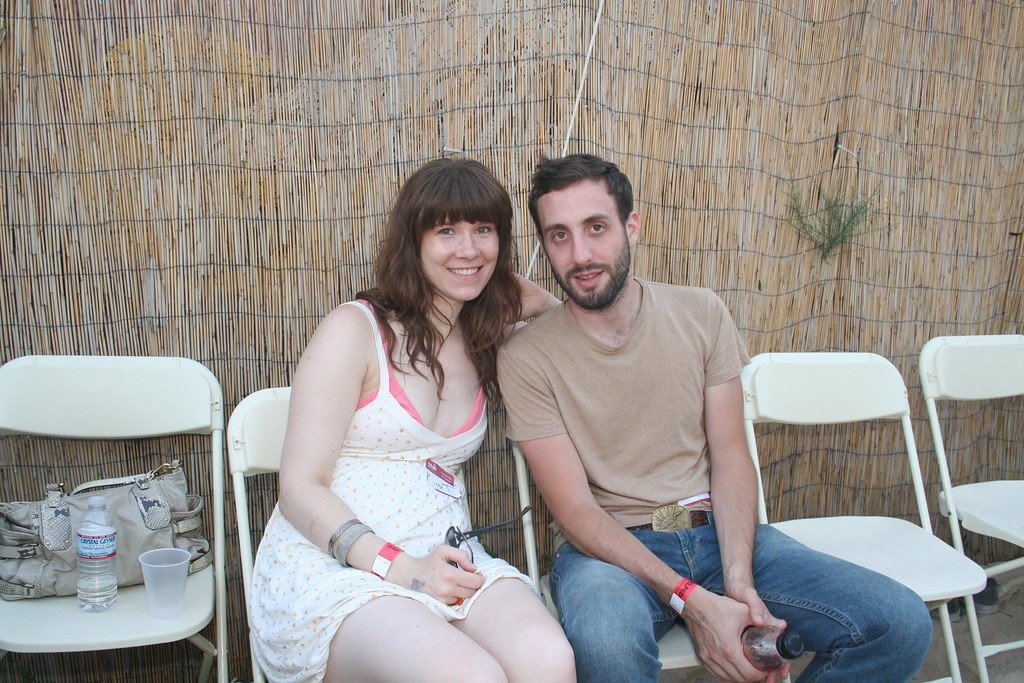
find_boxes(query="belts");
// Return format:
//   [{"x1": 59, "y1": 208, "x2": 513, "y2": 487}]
[{"x1": 626, "y1": 504, "x2": 708, "y2": 532}]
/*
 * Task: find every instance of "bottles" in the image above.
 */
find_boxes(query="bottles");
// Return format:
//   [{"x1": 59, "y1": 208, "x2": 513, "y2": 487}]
[
  {"x1": 78, "y1": 496, "x2": 117, "y2": 613},
  {"x1": 741, "y1": 625, "x2": 804, "y2": 672}
]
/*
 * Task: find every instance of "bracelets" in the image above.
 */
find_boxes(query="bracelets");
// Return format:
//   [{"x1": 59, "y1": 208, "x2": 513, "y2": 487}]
[
  {"x1": 669, "y1": 579, "x2": 698, "y2": 614},
  {"x1": 328, "y1": 518, "x2": 375, "y2": 567},
  {"x1": 370, "y1": 542, "x2": 405, "y2": 580}
]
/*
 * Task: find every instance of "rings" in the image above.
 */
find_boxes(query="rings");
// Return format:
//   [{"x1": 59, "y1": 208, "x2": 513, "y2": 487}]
[{"x1": 456, "y1": 597, "x2": 464, "y2": 606}]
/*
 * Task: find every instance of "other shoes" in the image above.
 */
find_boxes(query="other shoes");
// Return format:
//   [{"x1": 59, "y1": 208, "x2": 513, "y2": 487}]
[
  {"x1": 928, "y1": 596, "x2": 962, "y2": 623},
  {"x1": 968, "y1": 578, "x2": 999, "y2": 612}
]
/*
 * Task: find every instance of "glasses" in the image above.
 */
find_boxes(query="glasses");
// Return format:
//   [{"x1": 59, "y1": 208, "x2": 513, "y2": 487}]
[{"x1": 443, "y1": 505, "x2": 534, "y2": 570}]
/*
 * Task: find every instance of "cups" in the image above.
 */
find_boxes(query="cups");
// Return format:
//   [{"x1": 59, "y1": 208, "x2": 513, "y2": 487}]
[{"x1": 138, "y1": 548, "x2": 192, "y2": 619}]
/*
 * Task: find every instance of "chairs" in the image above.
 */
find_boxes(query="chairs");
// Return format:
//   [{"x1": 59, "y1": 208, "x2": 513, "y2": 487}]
[
  {"x1": 510, "y1": 437, "x2": 703, "y2": 669},
  {"x1": 0, "y1": 354, "x2": 230, "y2": 683},
  {"x1": 225, "y1": 385, "x2": 465, "y2": 683},
  {"x1": 740, "y1": 351, "x2": 987, "y2": 683},
  {"x1": 919, "y1": 333, "x2": 1024, "y2": 683}
]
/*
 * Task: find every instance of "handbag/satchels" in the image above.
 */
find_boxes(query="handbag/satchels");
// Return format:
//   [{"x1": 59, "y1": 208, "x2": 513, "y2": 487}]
[{"x1": 1, "y1": 459, "x2": 213, "y2": 602}]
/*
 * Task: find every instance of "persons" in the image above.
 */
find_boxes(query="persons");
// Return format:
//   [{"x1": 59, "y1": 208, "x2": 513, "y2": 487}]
[
  {"x1": 247, "y1": 156, "x2": 581, "y2": 683},
  {"x1": 493, "y1": 151, "x2": 933, "y2": 682}
]
